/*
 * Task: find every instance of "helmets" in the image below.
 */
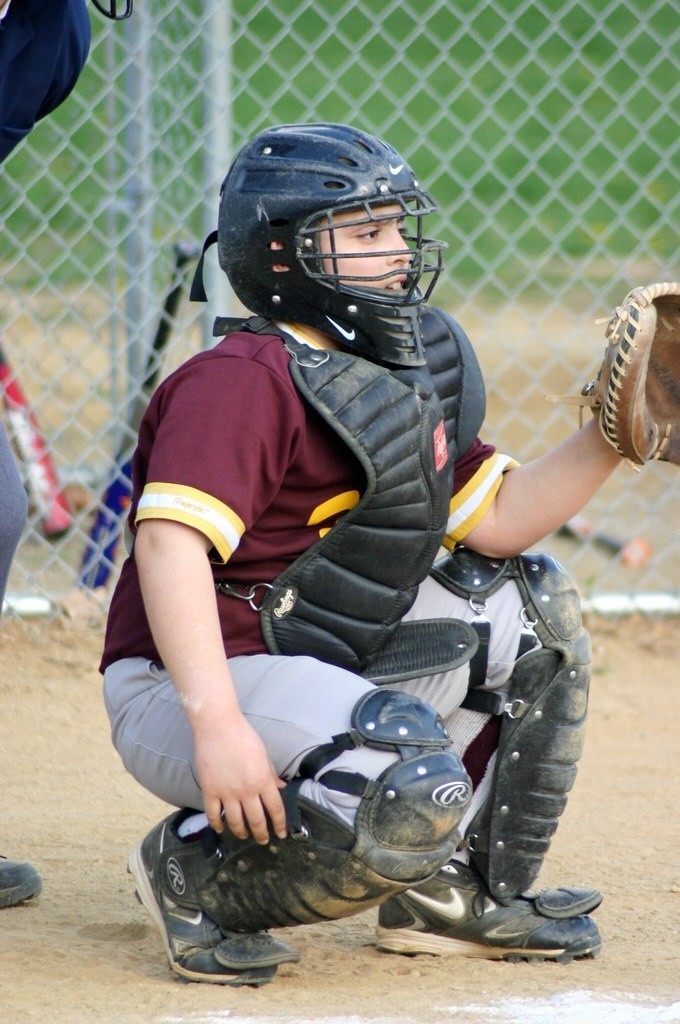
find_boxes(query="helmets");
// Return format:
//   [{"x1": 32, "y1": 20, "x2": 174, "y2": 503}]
[{"x1": 218, "y1": 125, "x2": 446, "y2": 367}]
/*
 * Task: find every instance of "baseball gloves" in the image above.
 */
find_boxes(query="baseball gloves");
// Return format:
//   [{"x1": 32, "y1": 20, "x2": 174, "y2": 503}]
[{"x1": 578, "y1": 281, "x2": 680, "y2": 467}]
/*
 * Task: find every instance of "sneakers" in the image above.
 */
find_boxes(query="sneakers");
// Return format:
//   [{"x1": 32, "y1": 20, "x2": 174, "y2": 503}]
[
  {"x1": 127, "y1": 806, "x2": 280, "y2": 984},
  {"x1": 376, "y1": 858, "x2": 604, "y2": 959}
]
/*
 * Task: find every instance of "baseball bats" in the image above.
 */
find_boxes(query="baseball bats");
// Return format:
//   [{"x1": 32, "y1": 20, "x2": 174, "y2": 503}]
[
  {"x1": 558, "y1": 526, "x2": 650, "y2": 566},
  {"x1": 79, "y1": 238, "x2": 196, "y2": 588},
  {"x1": 0, "y1": 341, "x2": 72, "y2": 544}
]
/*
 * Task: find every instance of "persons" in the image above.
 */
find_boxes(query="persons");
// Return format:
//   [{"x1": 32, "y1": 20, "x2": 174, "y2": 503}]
[
  {"x1": 0, "y1": 0, "x2": 91, "y2": 911},
  {"x1": 98, "y1": 123, "x2": 626, "y2": 989}
]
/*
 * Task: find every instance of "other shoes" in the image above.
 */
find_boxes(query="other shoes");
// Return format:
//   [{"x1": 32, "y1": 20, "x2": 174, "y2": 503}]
[{"x1": 0, "y1": 851, "x2": 40, "y2": 907}]
